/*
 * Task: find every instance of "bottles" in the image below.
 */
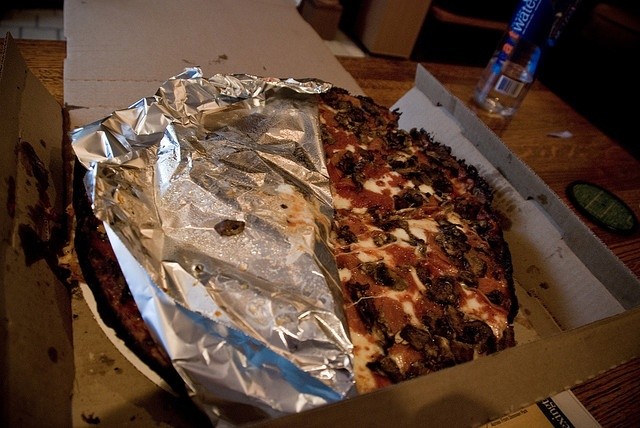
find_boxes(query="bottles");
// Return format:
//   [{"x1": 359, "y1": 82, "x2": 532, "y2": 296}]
[{"x1": 473, "y1": 0, "x2": 571, "y2": 121}]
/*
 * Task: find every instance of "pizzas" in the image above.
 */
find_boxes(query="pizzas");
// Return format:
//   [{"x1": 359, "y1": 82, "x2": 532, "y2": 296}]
[{"x1": 83, "y1": 77, "x2": 517, "y2": 400}]
